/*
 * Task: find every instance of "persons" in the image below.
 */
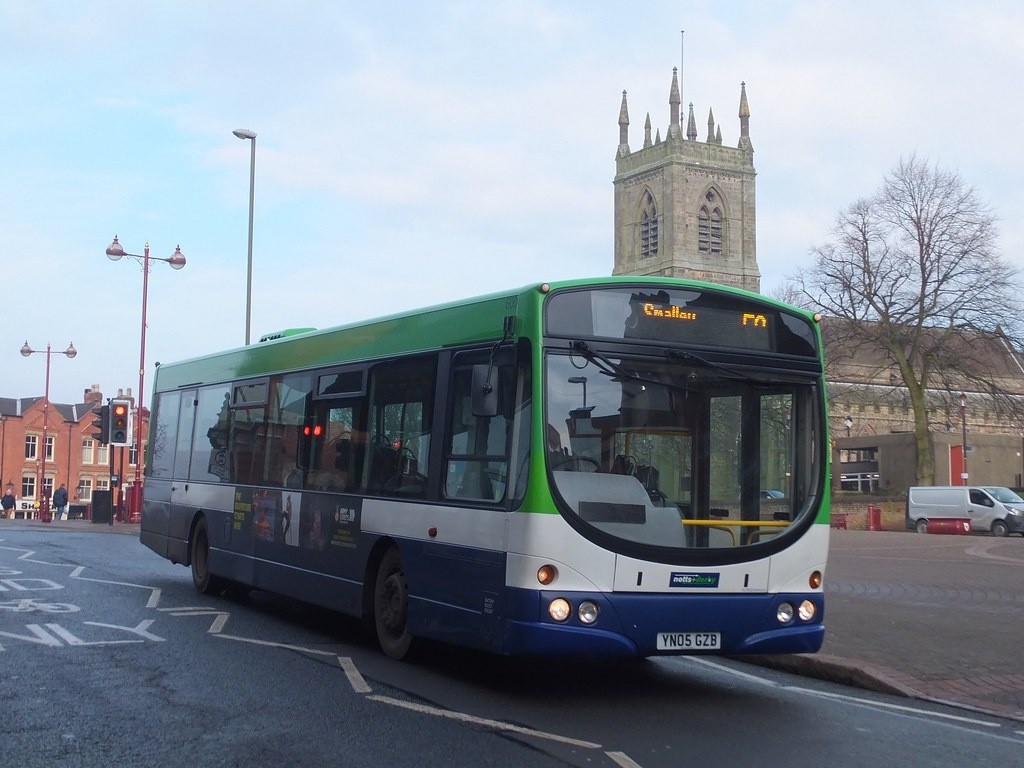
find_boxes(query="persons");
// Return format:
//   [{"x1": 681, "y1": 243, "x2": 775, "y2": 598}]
[
  {"x1": 281, "y1": 494, "x2": 292, "y2": 541},
  {"x1": 1, "y1": 489, "x2": 16, "y2": 519},
  {"x1": 515, "y1": 415, "x2": 587, "y2": 499},
  {"x1": 53, "y1": 483, "x2": 68, "y2": 519}
]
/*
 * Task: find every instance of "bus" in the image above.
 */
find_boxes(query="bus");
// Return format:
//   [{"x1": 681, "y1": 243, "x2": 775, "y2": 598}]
[{"x1": 140, "y1": 276, "x2": 831, "y2": 660}]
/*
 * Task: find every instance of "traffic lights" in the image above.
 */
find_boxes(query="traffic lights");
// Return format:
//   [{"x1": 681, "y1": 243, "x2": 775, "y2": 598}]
[
  {"x1": 108, "y1": 399, "x2": 134, "y2": 446},
  {"x1": 92, "y1": 404, "x2": 108, "y2": 444}
]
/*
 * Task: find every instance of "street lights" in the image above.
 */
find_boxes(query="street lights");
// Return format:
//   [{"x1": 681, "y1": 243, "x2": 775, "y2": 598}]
[
  {"x1": 233, "y1": 129, "x2": 257, "y2": 344},
  {"x1": 20, "y1": 340, "x2": 77, "y2": 518},
  {"x1": 844, "y1": 416, "x2": 853, "y2": 462},
  {"x1": 106, "y1": 235, "x2": 186, "y2": 524},
  {"x1": 961, "y1": 392, "x2": 967, "y2": 484}
]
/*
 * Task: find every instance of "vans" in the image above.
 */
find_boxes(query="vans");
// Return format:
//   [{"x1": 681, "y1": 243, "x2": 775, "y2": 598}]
[{"x1": 906, "y1": 485, "x2": 1024, "y2": 537}]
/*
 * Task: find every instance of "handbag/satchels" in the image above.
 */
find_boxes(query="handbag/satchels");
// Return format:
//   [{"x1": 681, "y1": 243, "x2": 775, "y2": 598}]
[{"x1": 63, "y1": 506, "x2": 68, "y2": 514}]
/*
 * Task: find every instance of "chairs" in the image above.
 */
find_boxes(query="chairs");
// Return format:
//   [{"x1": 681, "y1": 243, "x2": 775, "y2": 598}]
[{"x1": 176, "y1": 420, "x2": 419, "y2": 494}]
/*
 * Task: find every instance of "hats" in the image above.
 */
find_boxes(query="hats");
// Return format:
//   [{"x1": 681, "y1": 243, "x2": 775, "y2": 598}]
[{"x1": 6, "y1": 489, "x2": 11, "y2": 496}]
